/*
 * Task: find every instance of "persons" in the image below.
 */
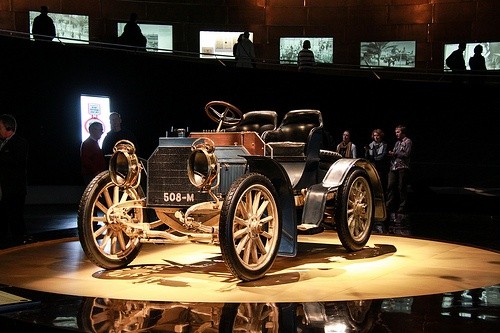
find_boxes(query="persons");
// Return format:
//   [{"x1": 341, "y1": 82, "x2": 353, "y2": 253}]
[
  {"x1": 371, "y1": 224, "x2": 411, "y2": 237},
  {"x1": 469, "y1": 45, "x2": 487, "y2": 70},
  {"x1": 298, "y1": 40, "x2": 316, "y2": 71},
  {"x1": 446, "y1": 42, "x2": 466, "y2": 72},
  {"x1": 32, "y1": 5, "x2": 56, "y2": 41},
  {"x1": 336, "y1": 129, "x2": 357, "y2": 159},
  {"x1": 364, "y1": 129, "x2": 389, "y2": 201},
  {"x1": 233, "y1": 32, "x2": 256, "y2": 68},
  {"x1": 111, "y1": 12, "x2": 147, "y2": 52},
  {"x1": 0, "y1": 114, "x2": 38, "y2": 249},
  {"x1": 81, "y1": 122, "x2": 104, "y2": 239},
  {"x1": 385, "y1": 125, "x2": 412, "y2": 222},
  {"x1": 102, "y1": 111, "x2": 138, "y2": 168}
]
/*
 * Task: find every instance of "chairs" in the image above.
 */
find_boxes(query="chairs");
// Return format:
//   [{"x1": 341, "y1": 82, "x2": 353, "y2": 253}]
[{"x1": 222, "y1": 109, "x2": 324, "y2": 162}]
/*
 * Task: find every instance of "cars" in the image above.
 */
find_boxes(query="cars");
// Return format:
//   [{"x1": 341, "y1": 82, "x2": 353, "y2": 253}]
[
  {"x1": 81, "y1": 297, "x2": 385, "y2": 333},
  {"x1": 77, "y1": 100, "x2": 388, "y2": 282}
]
